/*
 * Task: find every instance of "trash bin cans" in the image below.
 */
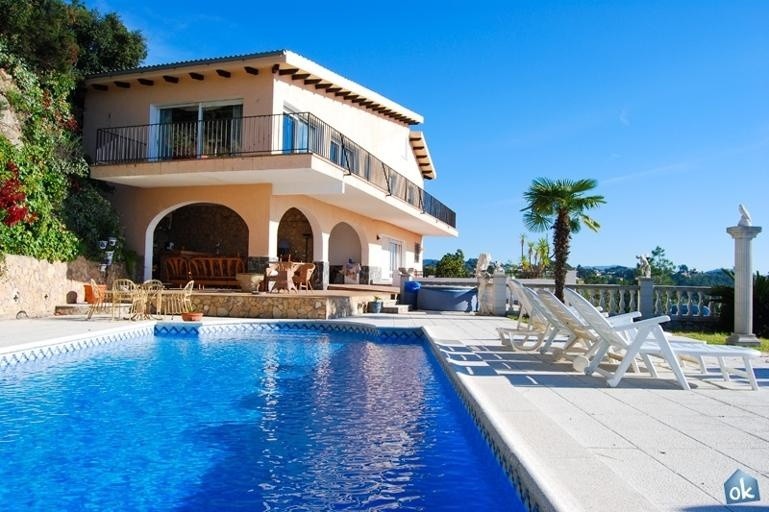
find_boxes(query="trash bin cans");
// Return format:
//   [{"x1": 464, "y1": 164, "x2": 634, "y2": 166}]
[{"x1": 400, "y1": 281, "x2": 421, "y2": 310}]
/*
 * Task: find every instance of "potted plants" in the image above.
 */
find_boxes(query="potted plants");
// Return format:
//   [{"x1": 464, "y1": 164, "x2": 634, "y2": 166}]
[{"x1": 368, "y1": 293, "x2": 383, "y2": 313}]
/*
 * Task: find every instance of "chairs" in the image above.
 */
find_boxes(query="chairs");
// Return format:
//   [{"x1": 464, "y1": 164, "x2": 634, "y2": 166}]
[
  {"x1": 496, "y1": 277, "x2": 761, "y2": 391},
  {"x1": 84, "y1": 260, "x2": 317, "y2": 321}
]
